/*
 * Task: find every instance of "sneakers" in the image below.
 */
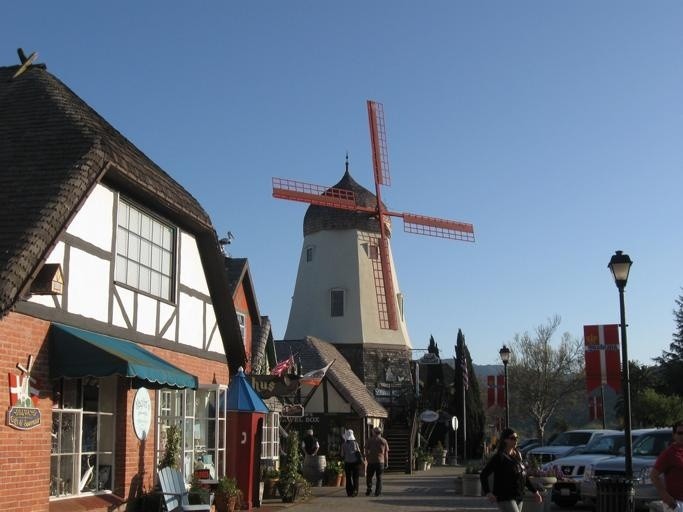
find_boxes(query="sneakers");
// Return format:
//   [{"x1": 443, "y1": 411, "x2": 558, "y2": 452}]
[{"x1": 346, "y1": 489, "x2": 382, "y2": 497}]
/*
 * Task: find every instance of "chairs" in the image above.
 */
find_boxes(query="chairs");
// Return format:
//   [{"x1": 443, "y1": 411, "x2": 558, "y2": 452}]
[{"x1": 152, "y1": 467, "x2": 215, "y2": 512}]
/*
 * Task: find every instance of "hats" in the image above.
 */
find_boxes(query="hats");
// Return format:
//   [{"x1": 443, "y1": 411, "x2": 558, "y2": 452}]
[
  {"x1": 370, "y1": 427, "x2": 382, "y2": 434},
  {"x1": 341, "y1": 429, "x2": 357, "y2": 441}
]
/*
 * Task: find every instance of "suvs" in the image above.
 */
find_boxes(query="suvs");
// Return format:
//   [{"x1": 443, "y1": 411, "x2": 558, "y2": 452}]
[{"x1": 514, "y1": 424, "x2": 674, "y2": 510}]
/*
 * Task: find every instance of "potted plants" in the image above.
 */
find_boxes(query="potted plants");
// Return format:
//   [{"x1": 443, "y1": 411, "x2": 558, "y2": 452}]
[
  {"x1": 215, "y1": 474, "x2": 241, "y2": 512},
  {"x1": 324, "y1": 461, "x2": 346, "y2": 487},
  {"x1": 460, "y1": 465, "x2": 481, "y2": 496},
  {"x1": 415, "y1": 447, "x2": 433, "y2": 471},
  {"x1": 278, "y1": 432, "x2": 310, "y2": 503},
  {"x1": 258, "y1": 466, "x2": 279, "y2": 505}
]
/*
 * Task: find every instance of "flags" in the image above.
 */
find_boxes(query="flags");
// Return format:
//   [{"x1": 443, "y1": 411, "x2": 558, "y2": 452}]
[
  {"x1": 270, "y1": 357, "x2": 290, "y2": 375},
  {"x1": 300, "y1": 360, "x2": 334, "y2": 386}
]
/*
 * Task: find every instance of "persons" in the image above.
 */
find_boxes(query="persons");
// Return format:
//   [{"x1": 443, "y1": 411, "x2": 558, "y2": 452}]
[
  {"x1": 300, "y1": 429, "x2": 320, "y2": 457},
  {"x1": 364, "y1": 427, "x2": 390, "y2": 497},
  {"x1": 480, "y1": 428, "x2": 543, "y2": 512},
  {"x1": 339, "y1": 430, "x2": 362, "y2": 497},
  {"x1": 649, "y1": 420, "x2": 683, "y2": 512}
]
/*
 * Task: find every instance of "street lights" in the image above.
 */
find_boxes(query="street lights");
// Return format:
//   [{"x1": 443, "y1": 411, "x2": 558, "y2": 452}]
[
  {"x1": 498, "y1": 342, "x2": 515, "y2": 434},
  {"x1": 605, "y1": 243, "x2": 639, "y2": 506}
]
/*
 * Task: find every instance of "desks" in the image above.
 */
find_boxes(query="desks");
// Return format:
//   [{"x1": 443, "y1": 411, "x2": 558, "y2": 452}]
[{"x1": 199, "y1": 479, "x2": 219, "y2": 484}]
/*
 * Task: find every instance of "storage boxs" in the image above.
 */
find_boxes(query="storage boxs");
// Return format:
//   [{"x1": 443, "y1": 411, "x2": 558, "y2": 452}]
[{"x1": 194, "y1": 469, "x2": 209, "y2": 479}]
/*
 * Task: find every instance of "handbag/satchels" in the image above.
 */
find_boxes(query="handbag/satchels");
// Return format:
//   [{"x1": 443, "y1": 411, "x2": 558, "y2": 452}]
[{"x1": 354, "y1": 450, "x2": 363, "y2": 464}]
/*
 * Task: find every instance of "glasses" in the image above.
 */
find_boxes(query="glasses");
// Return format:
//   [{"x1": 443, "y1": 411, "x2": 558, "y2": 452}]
[{"x1": 508, "y1": 437, "x2": 518, "y2": 441}]
